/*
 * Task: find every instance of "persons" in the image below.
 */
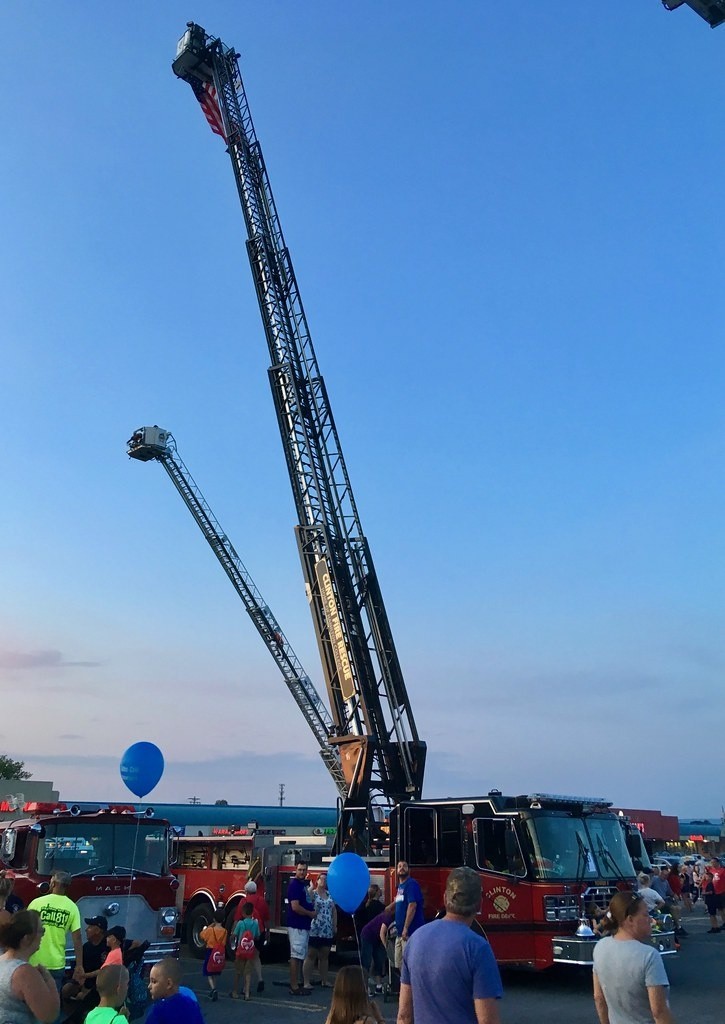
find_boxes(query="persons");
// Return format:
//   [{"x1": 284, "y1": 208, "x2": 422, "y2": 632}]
[
  {"x1": 592, "y1": 891, "x2": 673, "y2": 1024},
  {"x1": 200, "y1": 910, "x2": 228, "y2": 1000},
  {"x1": 637, "y1": 855, "x2": 725, "y2": 936},
  {"x1": 285, "y1": 861, "x2": 337, "y2": 996},
  {"x1": 230, "y1": 881, "x2": 269, "y2": 1001},
  {"x1": 354, "y1": 860, "x2": 424, "y2": 997},
  {"x1": 395, "y1": 867, "x2": 504, "y2": 1024},
  {"x1": 0, "y1": 868, "x2": 206, "y2": 1024},
  {"x1": 322, "y1": 963, "x2": 386, "y2": 1024}
]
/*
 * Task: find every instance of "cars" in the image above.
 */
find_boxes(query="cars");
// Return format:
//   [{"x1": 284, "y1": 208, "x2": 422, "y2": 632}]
[{"x1": 651, "y1": 851, "x2": 725, "y2": 872}]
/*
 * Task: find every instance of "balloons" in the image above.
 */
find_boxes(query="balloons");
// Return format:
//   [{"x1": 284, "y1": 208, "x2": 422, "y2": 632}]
[
  {"x1": 120, "y1": 742, "x2": 164, "y2": 799},
  {"x1": 327, "y1": 853, "x2": 371, "y2": 915}
]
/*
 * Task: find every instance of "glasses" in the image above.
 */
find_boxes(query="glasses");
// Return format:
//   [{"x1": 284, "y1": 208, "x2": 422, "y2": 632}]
[
  {"x1": 33, "y1": 928, "x2": 45, "y2": 935},
  {"x1": 624, "y1": 890, "x2": 641, "y2": 917}
]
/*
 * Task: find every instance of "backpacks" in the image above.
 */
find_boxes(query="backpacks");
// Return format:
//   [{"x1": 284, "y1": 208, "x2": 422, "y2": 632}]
[
  {"x1": 207, "y1": 928, "x2": 226, "y2": 972},
  {"x1": 236, "y1": 920, "x2": 255, "y2": 960}
]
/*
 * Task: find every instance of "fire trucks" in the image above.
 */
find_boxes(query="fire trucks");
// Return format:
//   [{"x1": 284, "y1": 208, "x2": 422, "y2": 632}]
[
  {"x1": 1, "y1": 799, "x2": 178, "y2": 976},
  {"x1": 123, "y1": 425, "x2": 655, "y2": 878},
  {"x1": 169, "y1": 20, "x2": 678, "y2": 993}
]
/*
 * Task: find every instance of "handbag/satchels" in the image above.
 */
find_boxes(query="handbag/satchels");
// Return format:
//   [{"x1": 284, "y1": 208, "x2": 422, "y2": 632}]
[
  {"x1": 253, "y1": 927, "x2": 271, "y2": 951},
  {"x1": 659, "y1": 914, "x2": 672, "y2": 931}
]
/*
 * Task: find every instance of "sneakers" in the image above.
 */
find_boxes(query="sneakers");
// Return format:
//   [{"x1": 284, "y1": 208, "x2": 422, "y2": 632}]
[{"x1": 206, "y1": 987, "x2": 218, "y2": 1001}]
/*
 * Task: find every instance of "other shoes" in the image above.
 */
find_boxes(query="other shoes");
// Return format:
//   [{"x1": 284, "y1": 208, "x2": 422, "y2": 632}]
[
  {"x1": 322, "y1": 980, "x2": 333, "y2": 987},
  {"x1": 303, "y1": 983, "x2": 314, "y2": 990},
  {"x1": 707, "y1": 927, "x2": 721, "y2": 933},
  {"x1": 257, "y1": 980, "x2": 264, "y2": 992},
  {"x1": 720, "y1": 923, "x2": 725, "y2": 930},
  {"x1": 288, "y1": 984, "x2": 312, "y2": 995},
  {"x1": 241, "y1": 995, "x2": 249, "y2": 1001},
  {"x1": 232, "y1": 994, "x2": 238, "y2": 999},
  {"x1": 368, "y1": 984, "x2": 384, "y2": 997},
  {"x1": 704, "y1": 909, "x2": 708, "y2": 914}
]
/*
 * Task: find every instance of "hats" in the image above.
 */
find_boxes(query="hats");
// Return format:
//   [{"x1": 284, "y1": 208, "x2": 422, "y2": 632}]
[
  {"x1": 85, "y1": 916, "x2": 126, "y2": 942},
  {"x1": 5, "y1": 870, "x2": 15, "y2": 880},
  {"x1": 445, "y1": 866, "x2": 482, "y2": 911},
  {"x1": 245, "y1": 881, "x2": 257, "y2": 892}
]
